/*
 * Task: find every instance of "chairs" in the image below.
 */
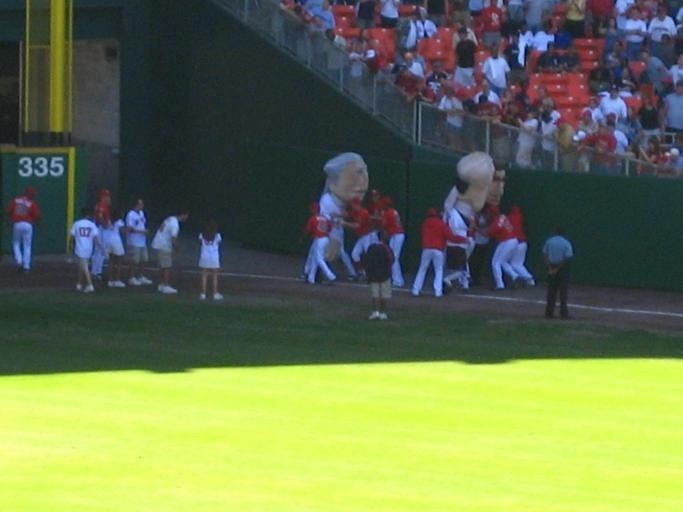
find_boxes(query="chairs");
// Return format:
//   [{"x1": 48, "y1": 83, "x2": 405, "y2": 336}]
[{"x1": 283, "y1": 1, "x2": 683, "y2": 175}]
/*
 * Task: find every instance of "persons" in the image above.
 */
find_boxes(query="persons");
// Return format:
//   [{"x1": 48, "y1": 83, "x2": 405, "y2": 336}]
[
  {"x1": 197, "y1": 220, "x2": 224, "y2": 300},
  {"x1": 66, "y1": 208, "x2": 106, "y2": 293},
  {"x1": 470, "y1": 161, "x2": 506, "y2": 289},
  {"x1": 319, "y1": 151, "x2": 369, "y2": 280},
  {"x1": 300, "y1": 189, "x2": 536, "y2": 321},
  {"x1": 443, "y1": 150, "x2": 495, "y2": 292},
  {"x1": 125, "y1": 199, "x2": 153, "y2": 286},
  {"x1": 151, "y1": 209, "x2": 189, "y2": 294},
  {"x1": 7, "y1": 186, "x2": 41, "y2": 273},
  {"x1": 89, "y1": 188, "x2": 112, "y2": 280},
  {"x1": 106, "y1": 207, "x2": 133, "y2": 287},
  {"x1": 281, "y1": 1, "x2": 682, "y2": 176},
  {"x1": 541, "y1": 225, "x2": 574, "y2": 320}
]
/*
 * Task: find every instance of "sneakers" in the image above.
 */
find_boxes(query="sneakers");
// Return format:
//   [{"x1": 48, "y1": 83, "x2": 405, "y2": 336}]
[
  {"x1": 107, "y1": 280, "x2": 125, "y2": 288},
  {"x1": 368, "y1": 311, "x2": 387, "y2": 320},
  {"x1": 157, "y1": 284, "x2": 179, "y2": 293},
  {"x1": 128, "y1": 276, "x2": 153, "y2": 287},
  {"x1": 77, "y1": 284, "x2": 94, "y2": 294},
  {"x1": 214, "y1": 293, "x2": 223, "y2": 300}
]
